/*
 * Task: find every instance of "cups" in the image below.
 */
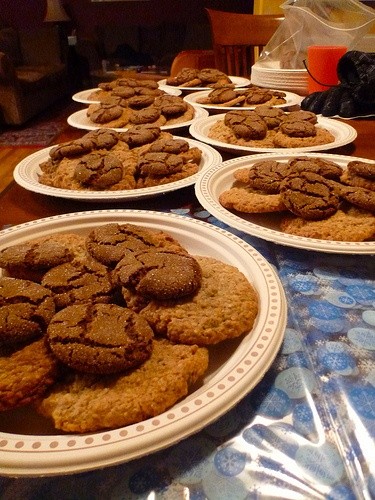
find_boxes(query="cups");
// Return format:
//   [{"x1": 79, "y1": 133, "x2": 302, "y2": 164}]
[{"x1": 302, "y1": 45, "x2": 348, "y2": 95}]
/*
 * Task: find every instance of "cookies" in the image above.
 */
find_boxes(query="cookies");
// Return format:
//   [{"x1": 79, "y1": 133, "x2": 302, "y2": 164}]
[
  {"x1": 219, "y1": 157, "x2": 375, "y2": 243},
  {"x1": 87, "y1": 78, "x2": 164, "y2": 100},
  {"x1": 86, "y1": 94, "x2": 195, "y2": 128},
  {"x1": 37, "y1": 123, "x2": 202, "y2": 191},
  {"x1": 0, "y1": 222, "x2": 259, "y2": 435},
  {"x1": 166, "y1": 68, "x2": 238, "y2": 89},
  {"x1": 207, "y1": 105, "x2": 335, "y2": 148},
  {"x1": 196, "y1": 84, "x2": 287, "y2": 108}
]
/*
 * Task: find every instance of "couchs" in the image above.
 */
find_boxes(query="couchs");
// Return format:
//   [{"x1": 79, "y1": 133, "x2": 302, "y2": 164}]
[{"x1": 0, "y1": 24, "x2": 69, "y2": 124}]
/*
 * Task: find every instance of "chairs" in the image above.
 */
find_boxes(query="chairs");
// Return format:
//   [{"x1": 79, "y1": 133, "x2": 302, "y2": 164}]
[
  {"x1": 169, "y1": 50, "x2": 226, "y2": 77},
  {"x1": 205, "y1": 8, "x2": 286, "y2": 78}
]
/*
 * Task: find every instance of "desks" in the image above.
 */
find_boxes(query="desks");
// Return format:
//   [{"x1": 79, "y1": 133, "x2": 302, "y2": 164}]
[
  {"x1": 0, "y1": 108, "x2": 375, "y2": 500},
  {"x1": 91, "y1": 67, "x2": 169, "y2": 83}
]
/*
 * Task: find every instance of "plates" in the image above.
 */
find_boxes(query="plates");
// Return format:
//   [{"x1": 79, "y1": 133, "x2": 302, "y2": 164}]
[
  {"x1": 71, "y1": 87, "x2": 182, "y2": 104},
  {"x1": 189, "y1": 112, "x2": 358, "y2": 154},
  {"x1": 12, "y1": 136, "x2": 222, "y2": 202},
  {"x1": 158, "y1": 75, "x2": 250, "y2": 93},
  {"x1": 194, "y1": 152, "x2": 375, "y2": 256},
  {"x1": 66, "y1": 105, "x2": 209, "y2": 133},
  {"x1": 0, "y1": 209, "x2": 288, "y2": 479},
  {"x1": 183, "y1": 88, "x2": 302, "y2": 112},
  {"x1": 251, "y1": 60, "x2": 309, "y2": 95}
]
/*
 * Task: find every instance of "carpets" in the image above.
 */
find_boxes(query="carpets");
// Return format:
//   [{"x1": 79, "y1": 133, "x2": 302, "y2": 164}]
[{"x1": 0, "y1": 99, "x2": 81, "y2": 149}]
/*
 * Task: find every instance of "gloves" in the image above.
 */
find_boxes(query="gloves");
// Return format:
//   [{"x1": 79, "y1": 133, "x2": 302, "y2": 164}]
[{"x1": 301, "y1": 85, "x2": 375, "y2": 118}]
